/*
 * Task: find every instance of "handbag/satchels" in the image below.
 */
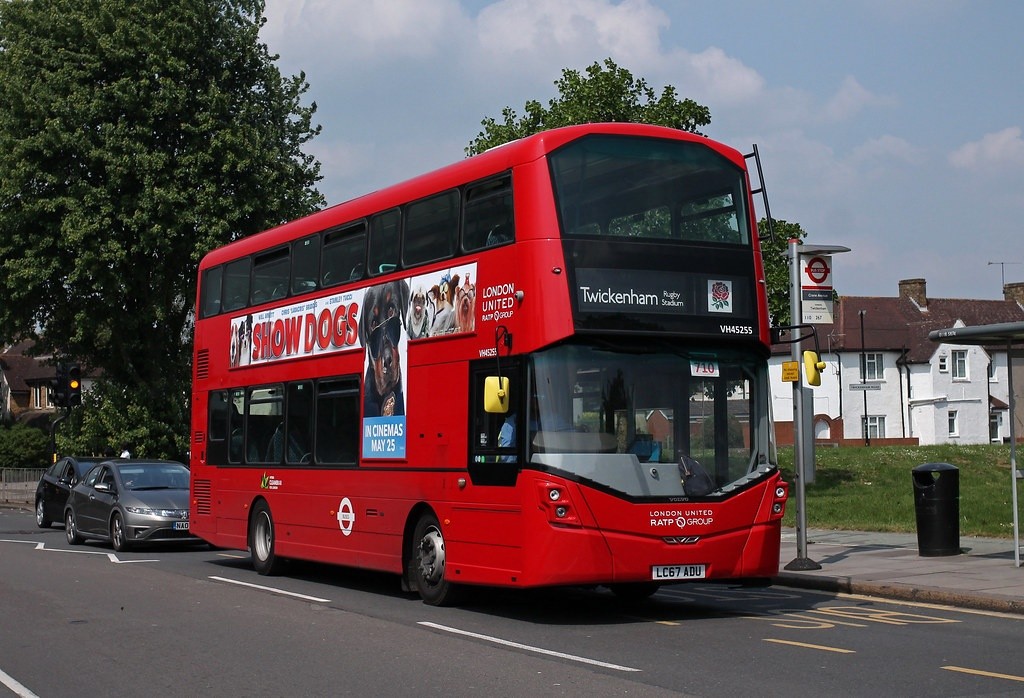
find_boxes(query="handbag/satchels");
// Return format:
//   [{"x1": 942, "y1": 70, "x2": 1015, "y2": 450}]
[{"x1": 678, "y1": 455, "x2": 715, "y2": 499}]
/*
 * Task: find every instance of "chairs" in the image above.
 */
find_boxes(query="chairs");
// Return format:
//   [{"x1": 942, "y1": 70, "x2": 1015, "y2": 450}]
[
  {"x1": 209, "y1": 225, "x2": 513, "y2": 314},
  {"x1": 210, "y1": 403, "x2": 356, "y2": 463},
  {"x1": 104, "y1": 475, "x2": 117, "y2": 491}
]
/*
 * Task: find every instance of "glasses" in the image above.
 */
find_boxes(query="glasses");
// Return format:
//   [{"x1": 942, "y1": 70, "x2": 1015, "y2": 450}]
[{"x1": 366, "y1": 315, "x2": 402, "y2": 358}]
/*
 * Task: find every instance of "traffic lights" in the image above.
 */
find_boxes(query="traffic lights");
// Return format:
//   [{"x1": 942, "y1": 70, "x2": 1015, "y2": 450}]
[
  {"x1": 65, "y1": 363, "x2": 81, "y2": 406},
  {"x1": 49, "y1": 364, "x2": 65, "y2": 406}
]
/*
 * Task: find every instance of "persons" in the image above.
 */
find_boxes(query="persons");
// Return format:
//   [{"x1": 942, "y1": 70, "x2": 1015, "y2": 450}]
[{"x1": 496, "y1": 393, "x2": 580, "y2": 462}]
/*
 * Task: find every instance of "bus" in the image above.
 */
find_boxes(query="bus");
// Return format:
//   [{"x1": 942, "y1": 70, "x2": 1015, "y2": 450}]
[{"x1": 189, "y1": 121, "x2": 827, "y2": 607}]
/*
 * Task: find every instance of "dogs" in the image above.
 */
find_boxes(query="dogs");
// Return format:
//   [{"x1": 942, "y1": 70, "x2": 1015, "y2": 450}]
[
  {"x1": 358, "y1": 278, "x2": 410, "y2": 418},
  {"x1": 230, "y1": 314, "x2": 253, "y2": 368},
  {"x1": 406, "y1": 274, "x2": 476, "y2": 339}
]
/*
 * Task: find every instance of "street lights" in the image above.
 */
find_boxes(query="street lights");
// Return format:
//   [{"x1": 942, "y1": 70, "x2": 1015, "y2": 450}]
[{"x1": 859, "y1": 309, "x2": 870, "y2": 445}]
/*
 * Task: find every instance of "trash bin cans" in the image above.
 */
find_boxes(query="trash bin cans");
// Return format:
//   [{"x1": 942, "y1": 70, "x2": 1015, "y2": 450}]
[{"x1": 911, "y1": 464, "x2": 960, "y2": 557}]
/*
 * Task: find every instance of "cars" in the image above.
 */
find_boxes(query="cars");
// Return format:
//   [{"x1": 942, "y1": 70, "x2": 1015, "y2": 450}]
[
  {"x1": 35, "y1": 456, "x2": 126, "y2": 528},
  {"x1": 64, "y1": 461, "x2": 218, "y2": 551}
]
